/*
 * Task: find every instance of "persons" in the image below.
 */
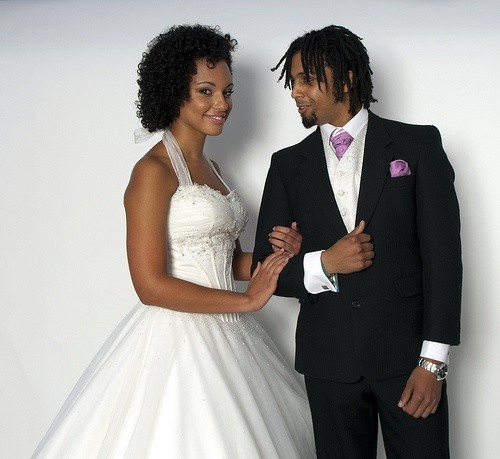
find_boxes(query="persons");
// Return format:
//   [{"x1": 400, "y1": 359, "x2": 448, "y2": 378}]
[
  {"x1": 31, "y1": 26, "x2": 319, "y2": 458},
  {"x1": 248, "y1": 24, "x2": 464, "y2": 459}
]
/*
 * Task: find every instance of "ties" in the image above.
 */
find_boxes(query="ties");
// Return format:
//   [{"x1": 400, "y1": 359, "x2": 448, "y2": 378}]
[{"x1": 331, "y1": 131, "x2": 354, "y2": 161}]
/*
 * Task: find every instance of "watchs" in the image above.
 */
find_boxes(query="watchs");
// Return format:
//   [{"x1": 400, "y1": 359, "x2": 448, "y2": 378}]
[{"x1": 417, "y1": 357, "x2": 449, "y2": 382}]
